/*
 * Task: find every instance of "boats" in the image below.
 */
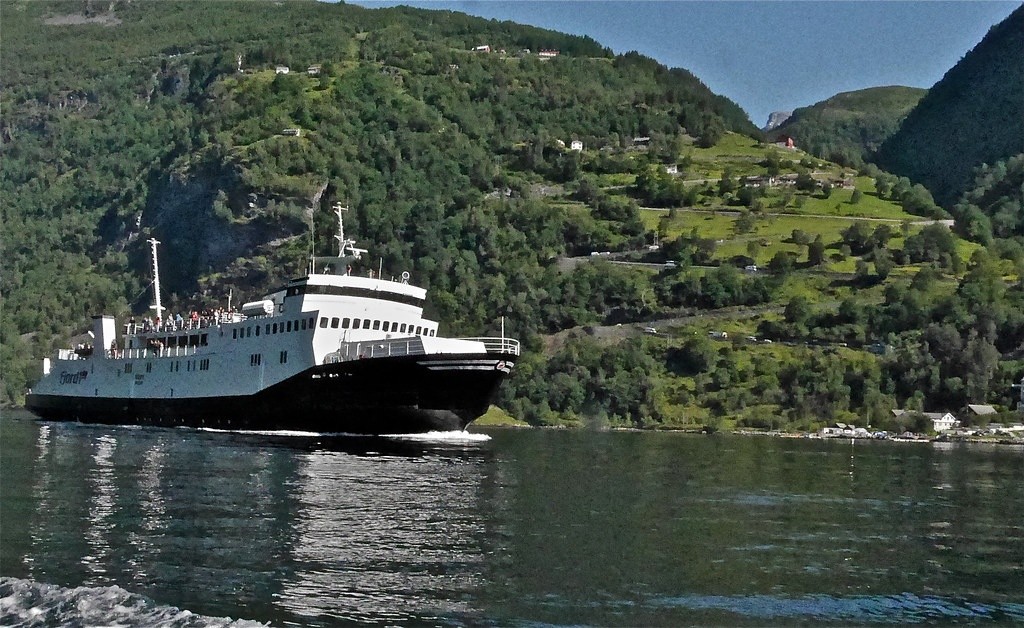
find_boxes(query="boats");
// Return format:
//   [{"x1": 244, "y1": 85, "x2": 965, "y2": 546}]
[
  {"x1": 74, "y1": 347, "x2": 93, "y2": 357},
  {"x1": 23, "y1": 201, "x2": 523, "y2": 434}
]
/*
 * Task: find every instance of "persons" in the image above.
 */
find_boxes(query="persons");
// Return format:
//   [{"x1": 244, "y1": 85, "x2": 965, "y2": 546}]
[
  {"x1": 111, "y1": 339, "x2": 118, "y2": 359},
  {"x1": 151, "y1": 340, "x2": 164, "y2": 358},
  {"x1": 110, "y1": 306, "x2": 237, "y2": 334}
]
